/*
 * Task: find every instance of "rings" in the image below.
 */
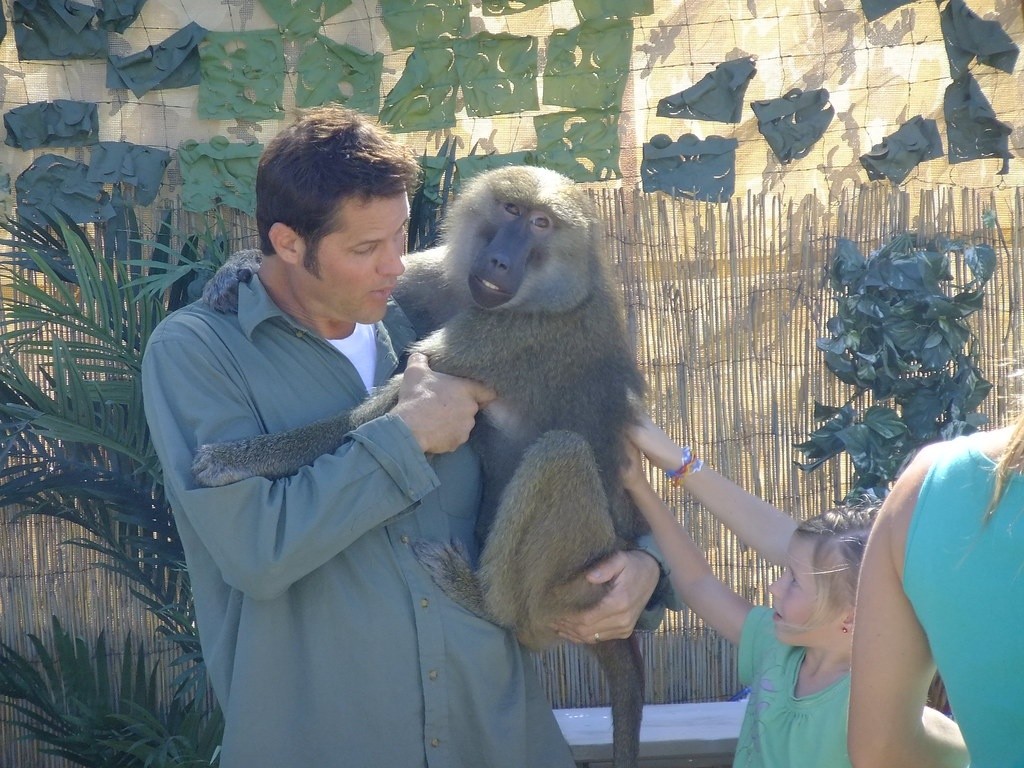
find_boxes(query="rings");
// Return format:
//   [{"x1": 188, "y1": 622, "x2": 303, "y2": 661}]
[{"x1": 594, "y1": 633, "x2": 601, "y2": 642}]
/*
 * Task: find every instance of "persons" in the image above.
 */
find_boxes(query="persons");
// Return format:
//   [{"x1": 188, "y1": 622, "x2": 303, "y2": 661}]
[
  {"x1": 616, "y1": 433, "x2": 883, "y2": 767},
  {"x1": 618, "y1": 383, "x2": 951, "y2": 716},
  {"x1": 144, "y1": 106, "x2": 686, "y2": 768},
  {"x1": 847, "y1": 405, "x2": 1024, "y2": 768}
]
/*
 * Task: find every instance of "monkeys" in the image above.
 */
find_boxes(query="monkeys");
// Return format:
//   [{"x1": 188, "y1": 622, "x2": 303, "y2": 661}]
[{"x1": 195, "y1": 164, "x2": 654, "y2": 768}]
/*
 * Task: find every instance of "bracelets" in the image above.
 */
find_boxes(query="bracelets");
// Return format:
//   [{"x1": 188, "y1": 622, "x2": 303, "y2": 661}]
[{"x1": 663, "y1": 445, "x2": 704, "y2": 488}]
